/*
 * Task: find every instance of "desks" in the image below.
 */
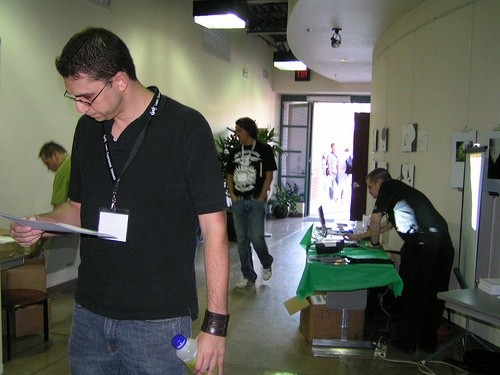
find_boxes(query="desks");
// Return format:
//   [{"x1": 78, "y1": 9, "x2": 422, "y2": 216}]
[
  {"x1": 297, "y1": 224, "x2": 405, "y2": 349},
  {"x1": 420, "y1": 287, "x2": 500, "y2": 365}
]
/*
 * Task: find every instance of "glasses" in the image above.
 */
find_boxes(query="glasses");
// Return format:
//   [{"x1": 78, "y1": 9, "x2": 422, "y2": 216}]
[{"x1": 64, "y1": 73, "x2": 118, "y2": 107}]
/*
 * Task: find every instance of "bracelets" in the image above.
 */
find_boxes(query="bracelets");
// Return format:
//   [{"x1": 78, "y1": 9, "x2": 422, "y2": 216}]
[
  {"x1": 200, "y1": 308, "x2": 227, "y2": 337},
  {"x1": 370, "y1": 241, "x2": 379, "y2": 246}
]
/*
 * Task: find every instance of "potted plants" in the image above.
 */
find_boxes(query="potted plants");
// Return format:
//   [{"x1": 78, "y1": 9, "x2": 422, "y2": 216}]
[
  {"x1": 268, "y1": 182, "x2": 301, "y2": 218},
  {"x1": 212, "y1": 126, "x2": 285, "y2": 242}
]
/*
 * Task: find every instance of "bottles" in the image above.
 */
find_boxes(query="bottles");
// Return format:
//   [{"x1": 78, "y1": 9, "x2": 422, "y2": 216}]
[{"x1": 171, "y1": 334, "x2": 218, "y2": 375}]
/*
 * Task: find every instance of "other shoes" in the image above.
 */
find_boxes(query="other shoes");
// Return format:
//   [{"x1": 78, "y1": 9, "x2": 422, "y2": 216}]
[
  {"x1": 238, "y1": 280, "x2": 254, "y2": 288},
  {"x1": 418, "y1": 342, "x2": 436, "y2": 354},
  {"x1": 262, "y1": 267, "x2": 272, "y2": 280},
  {"x1": 391, "y1": 339, "x2": 416, "y2": 354}
]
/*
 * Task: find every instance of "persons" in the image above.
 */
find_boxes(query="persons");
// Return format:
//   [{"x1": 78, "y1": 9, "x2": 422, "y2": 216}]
[
  {"x1": 227, "y1": 117, "x2": 278, "y2": 288},
  {"x1": 37, "y1": 140, "x2": 72, "y2": 212},
  {"x1": 366, "y1": 168, "x2": 454, "y2": 355},
  {"x1": 10, "y1": 25, "x2": 228, "y2": 375},
  {"x1": 322, "y1": 143, "x2": 353, "y2": 199}
]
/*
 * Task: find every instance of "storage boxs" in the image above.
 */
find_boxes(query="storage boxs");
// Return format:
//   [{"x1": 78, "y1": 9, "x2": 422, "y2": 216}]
[{"x1": 283, "y1": 290, "x2": 365, "y2": 346}]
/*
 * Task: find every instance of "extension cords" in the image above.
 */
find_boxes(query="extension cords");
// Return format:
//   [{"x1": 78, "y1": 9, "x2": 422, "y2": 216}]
[{"x1": 374, "y1": 343, "x2": 388, "y2": 358}]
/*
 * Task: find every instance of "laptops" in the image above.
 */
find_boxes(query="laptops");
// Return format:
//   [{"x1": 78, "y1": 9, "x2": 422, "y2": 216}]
[{"x1": 318, "y1": 205, "x2": 345, "y2": 236}]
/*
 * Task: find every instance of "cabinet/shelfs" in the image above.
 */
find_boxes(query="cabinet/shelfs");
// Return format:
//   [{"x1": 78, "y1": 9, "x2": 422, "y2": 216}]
[{"x1": 1, "y1": 229, "x2": 54, "y2": 362}]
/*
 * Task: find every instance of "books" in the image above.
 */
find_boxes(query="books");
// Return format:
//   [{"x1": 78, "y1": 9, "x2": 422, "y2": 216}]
[{"x1": 478, "y1": 277, "x2": 500, "y2": 296}]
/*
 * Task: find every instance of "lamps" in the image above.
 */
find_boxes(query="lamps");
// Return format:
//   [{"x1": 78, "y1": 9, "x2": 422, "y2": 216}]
[
  {"x1": 330, "y1": 27, "x2": 342, "y2": 48},
  {"x1": 194, "y1": 0, "x2": 247, "y2": 29},
  {"x1": 271, "y1": 51, "x2": 307, "y2": 71}
]
